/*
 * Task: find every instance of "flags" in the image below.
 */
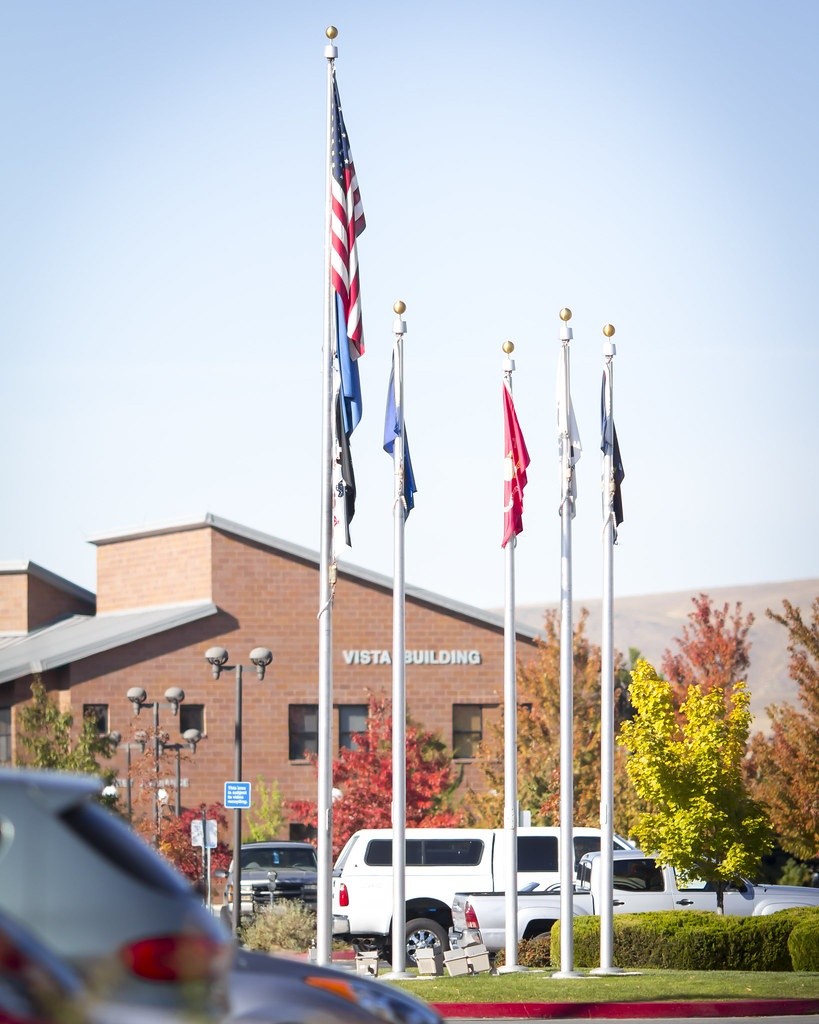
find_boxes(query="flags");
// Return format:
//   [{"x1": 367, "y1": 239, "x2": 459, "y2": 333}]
[
  {"x1": 384, "y1": 340, "x2": 417, "y2": 520},
  {"x1": 558, "y1": 346, "x2": 582, "y2": 520},
  {"x1": 601, "y1": 366, "x2": 625, "y2": 544},
  {"x1": 502, "y1": 380, "x2": 530, "y2": 547},
  {"x1": 331, "y1": 73, "x2": 367, "y2": 548}
]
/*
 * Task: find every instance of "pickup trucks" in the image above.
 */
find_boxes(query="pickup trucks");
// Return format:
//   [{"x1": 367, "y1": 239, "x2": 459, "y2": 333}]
[{"x1": 448, "y1": 849, "x2": 819, "y2": 953}]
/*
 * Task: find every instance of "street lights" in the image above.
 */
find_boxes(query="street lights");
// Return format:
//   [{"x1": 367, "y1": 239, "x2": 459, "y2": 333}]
[
  {"x1": 108, "y1": 730, "x2": 151, "y2": 825},
  {"x1": 204, "y1": 646, "x2": 273, "y2": 951},
  {"x1": 125, "y1": 686, "x2": 186, "y2": 856},
  {"x1": 133, "y1": 728, "x2": 203, "y2": 820}
]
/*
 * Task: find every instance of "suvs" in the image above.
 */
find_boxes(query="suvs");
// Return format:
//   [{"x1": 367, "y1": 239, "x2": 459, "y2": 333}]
[
  {"x1": 0, "y1": 766, "x2": 447, "y2": 1024},
  {"x1": 332, "y1": 826, "x2": 636, "y2": 970},
  {"x1": 214, "y1": 842, "x2": 319, "y2": 921}
]
